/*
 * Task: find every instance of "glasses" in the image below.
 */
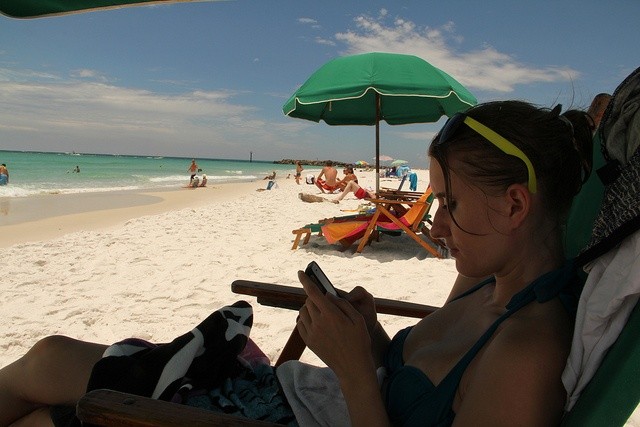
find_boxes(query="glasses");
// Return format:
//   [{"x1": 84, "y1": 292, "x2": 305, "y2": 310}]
[{"x1": 437, "y1": 112, "x2": 538, "y2": 193}]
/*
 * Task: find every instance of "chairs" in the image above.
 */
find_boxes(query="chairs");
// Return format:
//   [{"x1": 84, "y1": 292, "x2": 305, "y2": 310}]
[
  {"x1": 77, "y1": 92, "x2": 640, "y2": 427},
  {"x1": 291, "y1": 181, "x2": 449, "y2": 261}
]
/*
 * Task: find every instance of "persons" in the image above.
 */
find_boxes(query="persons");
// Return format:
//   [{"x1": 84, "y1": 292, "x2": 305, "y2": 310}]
[
  {"x1": 263, "y1": 171, "x2": 276, "y2": 180},
  {"x1": 313, "y1": 160, "x2": 338, "y2": 194},
  {"x1": 76, "y1": 165, "x2": 80, "y2": 172},
  {"x1": 191, "y1": 176, "x2": 199, "y2": 187},
  {"x1": 0, "y1": 163, "x2": 9, "y2": 186},
  {"x1": 199, "y1": 173, "x2": 208, "y2": 186},
  {"x1": 286, "y1": 173, "x2": 290, "y2": 179},
  {"x1": 0, "y1": 98, "x2": 596, "y2": 426},
  {"x1": 328, "y1": 179, "x2": 381, "y2": 204},
  {"x1": 337, "y1": 167, "x2": 349, "y2": 191},
  {"x1": 295, "y1": 161, "x2": 304, "y2": 184},
  {"x1": 336, "y1": 163, "x2": 359, "y2": 193},
  {"x1": 187, "y1": 159, "x2": 198, "y2": 185}
]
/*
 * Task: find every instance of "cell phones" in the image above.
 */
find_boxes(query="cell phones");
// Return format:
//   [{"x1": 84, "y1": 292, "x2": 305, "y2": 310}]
[{"x1": 306, "y1": 261, "x2": 338, "y2": 296}]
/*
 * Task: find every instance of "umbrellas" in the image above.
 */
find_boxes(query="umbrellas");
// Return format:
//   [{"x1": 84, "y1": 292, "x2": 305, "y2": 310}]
[
  {"x1": 390, "y1": 159, "x2": 409, "y2": 177},
  {"x1": 352, "y1": 160, "x2": 369, "y2": 174},
  {"x1": 282, "y1": 51, "x2": 477, "y2": 241},
  {"x1": 372, "y1": 154, "x2": 393, "y2": 173}
]
